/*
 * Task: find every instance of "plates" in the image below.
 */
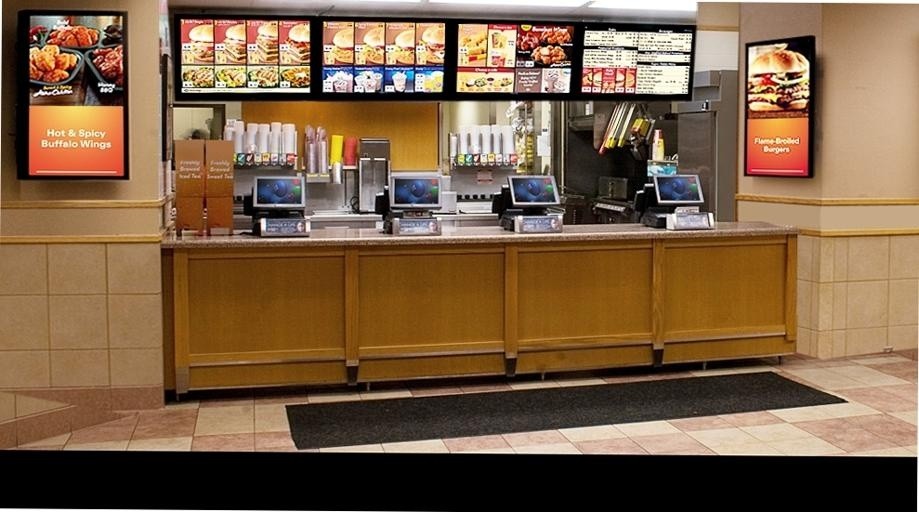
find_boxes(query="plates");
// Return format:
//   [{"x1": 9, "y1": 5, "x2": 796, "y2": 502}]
[
  {"x1": 29, "y1": 26, "x2": 125, "y2": 94},
  {"x1": 183, "y1": 67, "x2": 311, "y2": 89}
]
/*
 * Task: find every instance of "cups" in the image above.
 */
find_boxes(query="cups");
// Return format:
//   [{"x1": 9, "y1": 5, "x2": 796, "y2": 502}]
[
  {"x1": 224, "y1": 119, "x2": 298, "y2": 163},
  {"x1": 325, "y1": 68, "x2": 443, "y2": 93},
  {"x1": 303, "y1": 132, "x2": 358, "y2": 184}
]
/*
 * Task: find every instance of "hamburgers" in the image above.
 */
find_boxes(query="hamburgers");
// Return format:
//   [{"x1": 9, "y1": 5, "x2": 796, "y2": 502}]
[
  {"x1": 188, "y1": 21, "x2": 445, "y2": 67},
  {"x1": 746, "y1": 49, "x2": 810, "y2": 113}
]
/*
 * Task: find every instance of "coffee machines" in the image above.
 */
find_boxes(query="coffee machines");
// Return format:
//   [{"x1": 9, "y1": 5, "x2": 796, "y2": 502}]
[{"x1": 359, "y1": 136, "x2": 391, "y2": 212}]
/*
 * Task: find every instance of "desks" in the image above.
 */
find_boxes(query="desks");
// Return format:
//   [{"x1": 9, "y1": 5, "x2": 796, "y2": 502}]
[{"x1": 160, "y1": 221, "x2": 799, "y2": 402}]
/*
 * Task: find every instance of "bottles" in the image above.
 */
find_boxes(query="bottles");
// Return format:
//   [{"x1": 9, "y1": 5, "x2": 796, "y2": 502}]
[{"x1": 652, "y1": 129, "x2": 665, "y2": 162}]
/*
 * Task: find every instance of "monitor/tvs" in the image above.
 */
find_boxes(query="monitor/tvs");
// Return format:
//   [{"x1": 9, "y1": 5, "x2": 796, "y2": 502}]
[
  {"x1": 243, "y1": 186, "x2": 289, "y2": 218},
  {"x1": 654, "y1": 174, "x2": 705, "y2": 206},
  {"x1": 635, "y1": 183, "x2": 673, "y2": 214},
  {"x1": 506, "y1": 175, "x2": 561, "y2": 208},
  {"x1": 389, "y1": 173, "x2": 443, "y2": 209},
  {"x1": 375, "y1": 185, "x2": 403, "y2": 220},
  {"x1": 490, "y1": 185, "x2": 547, "y2": 220},
  {"x1": 253, "y1": 176, "x2": 306, "y2": 210}
]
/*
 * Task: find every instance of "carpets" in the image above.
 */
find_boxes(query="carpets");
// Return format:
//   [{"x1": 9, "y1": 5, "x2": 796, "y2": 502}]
[{"x1": 282, "y1": 370, "x2": 851, "y2": 449}]
